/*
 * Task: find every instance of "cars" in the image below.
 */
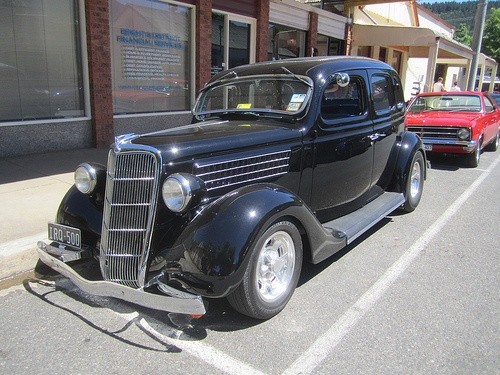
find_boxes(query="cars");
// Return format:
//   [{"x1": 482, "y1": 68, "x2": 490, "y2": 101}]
[
  {"x1": 467, "y1": 93, "x2": 500, "y2": 106},
  {"x1": 35, "y1": 54, "x2": 432, "y2": 318},
  {"x1": 51, "y1": 70, "x2": 191, "y2": 118},
  {"x1": 0, "y1": 64, "x2": 60, "y2": 122},
  {"x1": 404, "y1": 91, "x2": 500, "y2": 166}
]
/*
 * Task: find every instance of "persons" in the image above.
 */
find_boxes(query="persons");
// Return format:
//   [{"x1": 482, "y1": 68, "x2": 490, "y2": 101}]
[
  {"x1": 451, "y1": 81, "x2": 460, "y2": 91},
  {"x1": 433, "y1": 77, "x2": 443, "y2": 92}
]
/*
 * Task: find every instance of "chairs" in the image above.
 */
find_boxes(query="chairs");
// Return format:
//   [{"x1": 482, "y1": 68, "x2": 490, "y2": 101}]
[
  {"x1": 411, "y1": 104, "x2": 493, "y2": 114},
  {"x1": 274, "y1": 93, "x2": 362, "y2": 121}
]
